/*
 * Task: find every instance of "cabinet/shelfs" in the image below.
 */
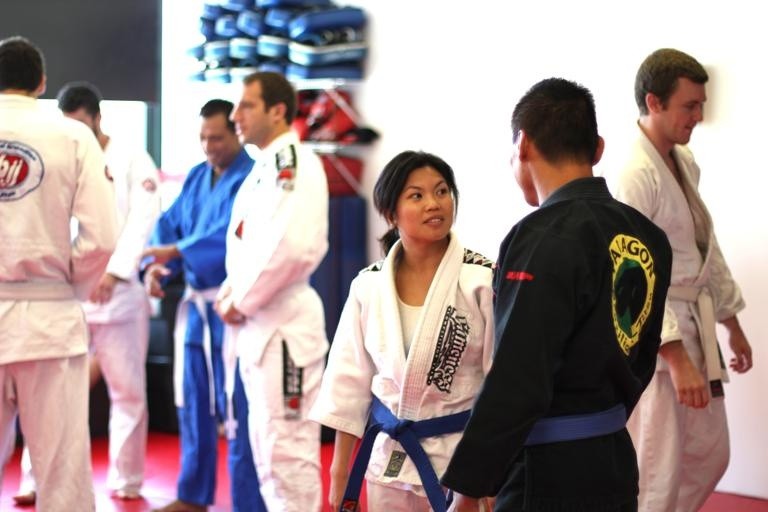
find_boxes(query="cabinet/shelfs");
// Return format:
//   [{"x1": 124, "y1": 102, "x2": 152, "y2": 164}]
[{"x1": 290, "y1": 76, "x2": 371, "y2": 204}]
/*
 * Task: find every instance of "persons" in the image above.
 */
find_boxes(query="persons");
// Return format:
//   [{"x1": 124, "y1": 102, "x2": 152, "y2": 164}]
[
  {"x1": 12, "y1": 81, "x2": 162, "y2": 504},
  {"x1": 307, "y1": 152, "x2": 498, "y2": 511},
  {"x1": 213, "y1": 72, "x2": 330, "y2": 510},
  {"x1": 610, "y1": 47, "x2": 752, "y2": 511},
  {"x1": 440, "y1": 77, "x2": 673, "y2": 511},
  {"x1": 0, "y1": 34, "x2": 122, "y2": 510},
  {"x1": 137, "y1": 97, "x2": 255, "y2": 512}
]
